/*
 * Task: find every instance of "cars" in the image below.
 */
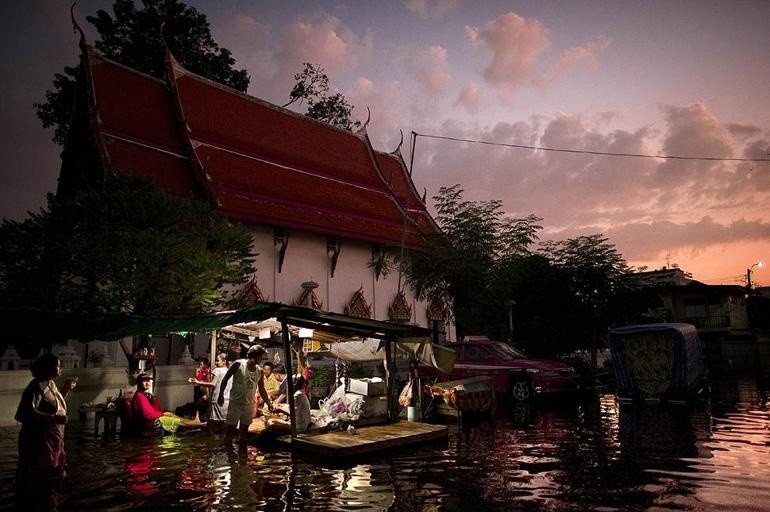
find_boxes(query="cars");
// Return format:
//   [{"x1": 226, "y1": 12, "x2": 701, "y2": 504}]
[{"x1": 414, "y1": 333, "x2": 587, "y2": 403}]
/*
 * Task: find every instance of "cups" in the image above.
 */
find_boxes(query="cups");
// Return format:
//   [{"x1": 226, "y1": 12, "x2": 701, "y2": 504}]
[
  {"x1": 66, "y1": 376, "x2": 80, "y2": 390},
  {"x1": 107, "y1": 396, "x2": 112, "y2": 404},
  {"x1": 407, "y1": 407, "x2": 415, "y2": 423}
]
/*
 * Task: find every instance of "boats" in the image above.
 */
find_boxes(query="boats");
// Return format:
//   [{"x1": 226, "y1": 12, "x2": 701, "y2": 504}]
[{"x1": 607, "y1": 322, "x2": 713, "y2": 409}]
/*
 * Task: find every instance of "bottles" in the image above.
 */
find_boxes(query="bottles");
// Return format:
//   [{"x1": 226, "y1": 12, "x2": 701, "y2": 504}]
[{"x1": 117, "y1": 389, "x2": 124, "y2": 407}]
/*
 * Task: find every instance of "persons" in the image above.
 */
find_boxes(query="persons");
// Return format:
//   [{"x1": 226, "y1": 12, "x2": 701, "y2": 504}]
[
  {"x1": 216, "y1": 343, "x2": 274, "y2": 448},
  {"x1": 194, "y1": 352, "x2": 312, "y2": 448},
  {"x1": 224, "y1": 445, "x2": 258, "y2": 512},
  {"x1": 130, "y1": 373, "x2": 203, "y2": 435},
  {"x1": 14, "y1": 351, "x2": 80, "y2": 480}
]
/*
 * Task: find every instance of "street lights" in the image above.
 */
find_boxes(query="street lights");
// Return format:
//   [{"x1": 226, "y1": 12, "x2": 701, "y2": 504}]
[{"x1": 746, "y1": 261, "x2": 765, "y2": 340}]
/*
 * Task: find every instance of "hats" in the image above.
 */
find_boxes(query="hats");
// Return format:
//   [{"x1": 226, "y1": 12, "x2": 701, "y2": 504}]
[{"x1": 135, "y1": 372, "x2": 155, "y2": 384}]
